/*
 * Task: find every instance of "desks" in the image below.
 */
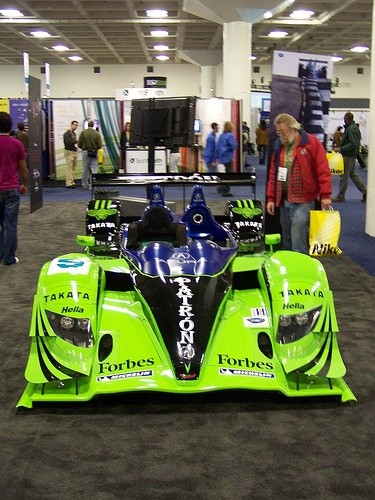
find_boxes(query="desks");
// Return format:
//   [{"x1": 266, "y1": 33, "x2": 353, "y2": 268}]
[{"x1": 87, "y1": 166, "x2": 257, "y2": 202}]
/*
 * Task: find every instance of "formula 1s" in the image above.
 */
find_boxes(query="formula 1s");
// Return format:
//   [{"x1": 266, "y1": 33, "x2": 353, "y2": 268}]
[{"x1": 16, "y1": 187, "x2": 357, "y2": 408}]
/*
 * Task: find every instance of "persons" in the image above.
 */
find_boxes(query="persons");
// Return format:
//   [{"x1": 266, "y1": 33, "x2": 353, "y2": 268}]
[
  {"x1": 204, "y1": 123, "x2": 219, "y2": 173},
  {"x1": 120, "y1": 122, "x2": 131, "y2": 160},
  {"x1": 78, "y1": 121, "x2": 102, "y2": 191},
  {"x1": 0, "y1": 111, "x2": 29, "y2": 265},
  {"x1": 216, "y1": 121, "x2": 239, "y2": 197},
  {"x1": 267, "y1": 113, "x2": 332, "y2": 256},
  {"x1": 63, "y1": 121, "x2": 78, "y2": 189},
  {"x1": 332, "y1": 112, "x2": 367, "y2": 203},
  {"x1": 242, "y1": 119, "x2": 270, "y2": 167}
]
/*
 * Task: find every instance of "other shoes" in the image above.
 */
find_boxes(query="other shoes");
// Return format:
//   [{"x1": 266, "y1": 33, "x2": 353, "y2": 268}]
[
  {"x1": 332, "y1": 197, "x2": 345, "y2": 203},
  {"x1": 65, "y1": 186, "x2": 76, "y2": 189},
  {"x1": 361, "y1": 198, "x2": 365, "y2": 203},
  {"x1": 359, "y1": 166, "x2": 366, "y2": 168},
  {"x1": 4, "y1": 257, "x2": 19, "y2": 265},
  {"x1": 221, "y1": 194, "x2": 234, "y2": 197}
]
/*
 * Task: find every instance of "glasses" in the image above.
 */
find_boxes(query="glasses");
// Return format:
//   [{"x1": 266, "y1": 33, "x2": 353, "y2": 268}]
[{"x1": 276, "y1": 130, "x2": 289, "y2": 135}]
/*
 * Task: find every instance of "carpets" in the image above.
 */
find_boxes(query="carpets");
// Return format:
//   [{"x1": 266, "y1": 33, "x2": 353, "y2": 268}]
[{"x1": 0, "y1": 200, "x2": 375, "y2": 500}]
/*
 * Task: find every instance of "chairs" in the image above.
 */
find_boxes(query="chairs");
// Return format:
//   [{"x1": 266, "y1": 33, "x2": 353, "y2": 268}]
[
  {"x1": 121, "y1": 185, "x2": 188, "y2": 250},
  {"x1": 179, "y1": 184, "x2": 227, "y2": 244}
]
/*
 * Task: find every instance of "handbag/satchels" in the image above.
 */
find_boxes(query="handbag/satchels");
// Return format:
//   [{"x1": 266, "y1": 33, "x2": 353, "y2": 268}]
[
  {"x1": 309, "y1": 204, "x2": 343, "y2": 256},
  {"x1": 326, "y1": 150, "x2": 344, "y2": 176},
  {"x1": 87, "y1": 147, "x2": 97, "y2": 158}
]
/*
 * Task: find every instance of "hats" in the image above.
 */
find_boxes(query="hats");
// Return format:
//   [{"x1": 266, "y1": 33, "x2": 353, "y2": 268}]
[{"x1": 88, "y1": 119, "x2": 94, "y2": 124}]
